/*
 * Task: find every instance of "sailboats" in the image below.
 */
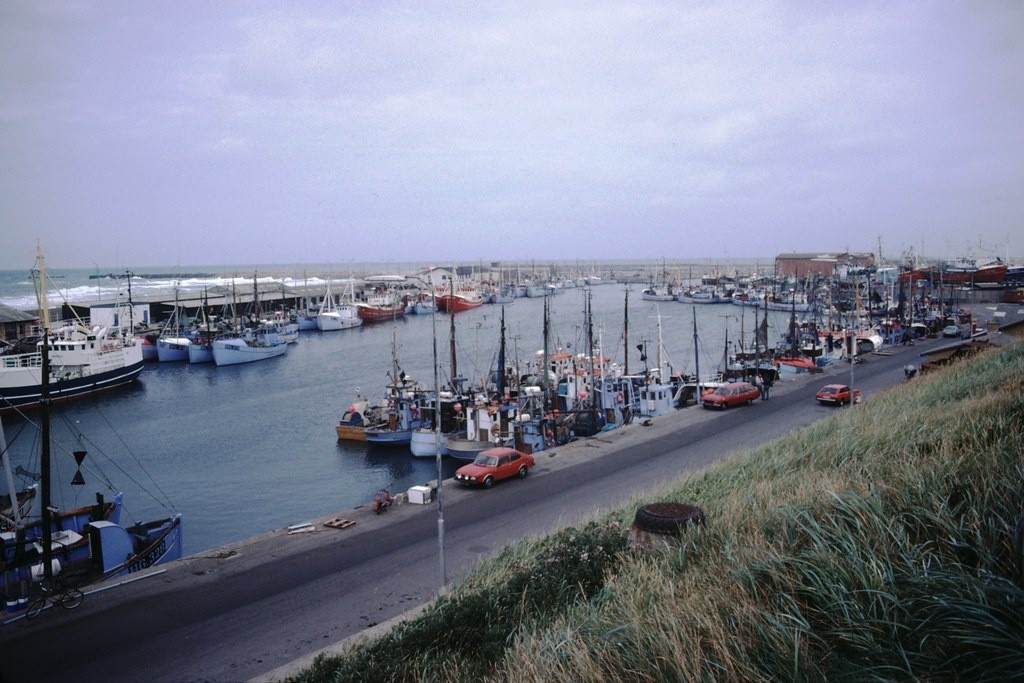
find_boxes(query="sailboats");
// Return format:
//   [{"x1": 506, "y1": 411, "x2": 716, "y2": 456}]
[{"x1": 0, "y1": 236, "x2": 1024, "y2": 610}]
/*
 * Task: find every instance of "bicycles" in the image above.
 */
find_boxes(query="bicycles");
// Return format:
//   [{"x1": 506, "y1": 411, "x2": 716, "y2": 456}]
[{"x1": 25, "y1": 580, "x2": 86, "y2": 619}]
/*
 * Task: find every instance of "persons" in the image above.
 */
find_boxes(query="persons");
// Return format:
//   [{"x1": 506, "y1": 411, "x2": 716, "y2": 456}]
[{"x1": 755, "y1": 372, "x2": 770, "y2": 400}]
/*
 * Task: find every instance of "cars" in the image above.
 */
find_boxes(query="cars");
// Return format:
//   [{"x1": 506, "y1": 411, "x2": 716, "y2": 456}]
[
  {"x1": 942, "y1": 325, "x2": 961, "y2": 338},
  {"x1": 453, "y1": 447, "x2": 534, "y2": 490},
  {"x1": 703, "y1": 381, "x2": 761, "y2": 411},
  {"x1": 815, "y1": 384, "x2": 861, "y2": 407}
]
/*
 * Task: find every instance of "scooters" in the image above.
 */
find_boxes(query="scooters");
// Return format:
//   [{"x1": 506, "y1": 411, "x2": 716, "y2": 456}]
[{"x1": 374, "y1": 482, "x2": 393, "y2": 515}]
